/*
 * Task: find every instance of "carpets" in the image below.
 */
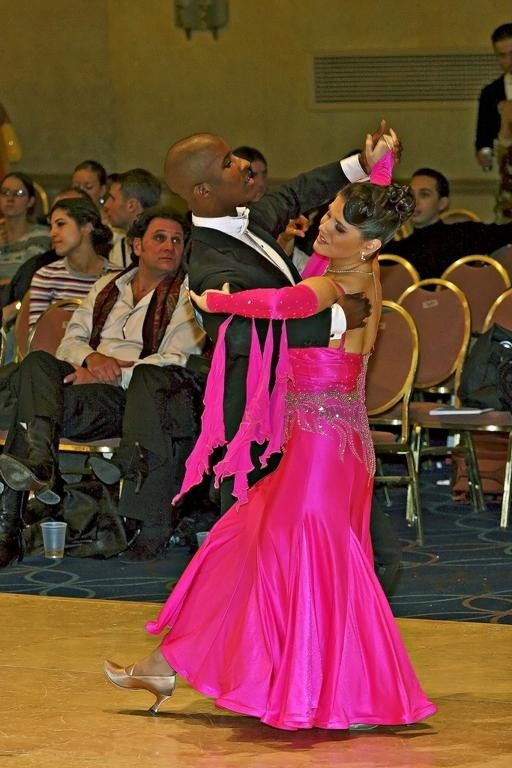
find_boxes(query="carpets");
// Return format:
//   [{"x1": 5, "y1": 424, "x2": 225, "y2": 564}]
[{"x1": 0, "y1": 460, "x2": 512, "y2": 624}]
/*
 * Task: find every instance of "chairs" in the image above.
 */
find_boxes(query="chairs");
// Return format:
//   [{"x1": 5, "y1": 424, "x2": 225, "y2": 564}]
[
  {"x1": 0, "y1": 288, "x2": 121, "y2": 564},
  {"x1": 364, "y1": 208, "x2": 511, "y2": 548}
]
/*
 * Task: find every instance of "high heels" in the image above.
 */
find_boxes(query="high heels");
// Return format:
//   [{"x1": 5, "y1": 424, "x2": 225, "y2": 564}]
[
  {"x1": 89, "y1": 442, "x2": 149, "y2": 494},
  {"x1": 103, "y1": 659, "x2": 177, "y2": 715},
  {"x1": 119, "y1": 534, "x2": 169, "y2": 564}
]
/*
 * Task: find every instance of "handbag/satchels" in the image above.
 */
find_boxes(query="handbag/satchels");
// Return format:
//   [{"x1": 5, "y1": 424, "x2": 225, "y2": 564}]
[{"x1": 458, "y1": 323, "x2": 512, "y2": 409}]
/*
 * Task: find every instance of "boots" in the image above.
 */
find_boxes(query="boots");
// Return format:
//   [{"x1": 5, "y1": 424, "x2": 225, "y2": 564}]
[
  {"x1": 0, "y1": 416, "x2": 63, "y2": 505},
  {"x1": 0, "y1": 485, "x2": 29, "y2": 569}
]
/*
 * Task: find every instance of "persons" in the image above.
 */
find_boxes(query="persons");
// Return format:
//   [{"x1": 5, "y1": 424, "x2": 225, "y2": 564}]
[
  {"x1": 165, "y1": 117, "x2": 404, "y2": 519},
  {"x1": 233, "y1": 146, "x2": 512, "y2": 467},
  {"x1": 470, "y1": 23, "x2": 511, "y2": 173},
  {"x1": 0, "y1": 161, "x2": 208, "y2": 568},
  {"x1": 99, "y1": 127, "x2": 439, "y2": 732}
]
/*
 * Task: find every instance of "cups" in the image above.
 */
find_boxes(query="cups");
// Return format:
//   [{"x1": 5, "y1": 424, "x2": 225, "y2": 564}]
[{"x1": 39, "y1": 521, "x2": 68, "y2": 560}]
